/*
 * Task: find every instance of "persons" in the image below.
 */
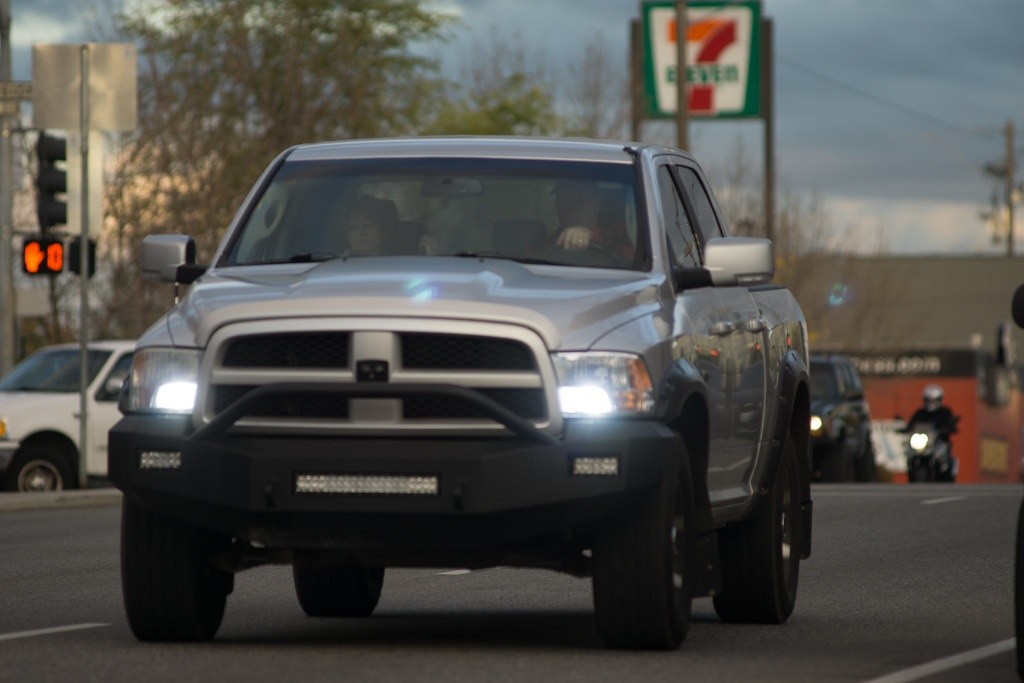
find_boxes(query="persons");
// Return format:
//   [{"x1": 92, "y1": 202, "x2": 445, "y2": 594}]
[
  {"x1": 903, "y1": 385, "x2": 957, "y2": 479},
  {"x1": 550, "y1": 200, "x2": 633, "y2": 259},
  {"x1": 343, "y1": 196, "x2": 440, "y2": 255}
]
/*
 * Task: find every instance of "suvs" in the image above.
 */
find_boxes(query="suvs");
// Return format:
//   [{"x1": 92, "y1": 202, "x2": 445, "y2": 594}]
[{"x1": 808, "y1": 358, "x2": 875, "y2": 482}]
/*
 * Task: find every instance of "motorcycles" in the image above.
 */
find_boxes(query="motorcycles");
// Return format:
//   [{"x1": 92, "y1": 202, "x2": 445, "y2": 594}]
[{"x1": 893, "y1": 413, "x2": 960, "y2": 481}]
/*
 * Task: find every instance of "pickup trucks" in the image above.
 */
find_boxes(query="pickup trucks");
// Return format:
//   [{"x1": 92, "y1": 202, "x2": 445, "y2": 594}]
[{"x1": 106, "y1": 135, "x2": 817, "y2": 652}]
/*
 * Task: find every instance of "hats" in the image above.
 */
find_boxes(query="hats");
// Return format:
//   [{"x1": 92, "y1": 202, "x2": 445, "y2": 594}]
[{"x1": 555, "y1": 177, "x2": 598, "y2": 201}]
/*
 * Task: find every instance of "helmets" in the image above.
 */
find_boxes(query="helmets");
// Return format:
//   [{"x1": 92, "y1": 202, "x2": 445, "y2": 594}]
[{"x1": 923, "y1": 384, "x2": 943, "y2": 400}]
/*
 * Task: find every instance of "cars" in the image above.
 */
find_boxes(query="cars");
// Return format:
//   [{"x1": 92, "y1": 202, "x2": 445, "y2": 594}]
[{"x1": 2, "y1": 343, "x2": 136, "y2": 494}]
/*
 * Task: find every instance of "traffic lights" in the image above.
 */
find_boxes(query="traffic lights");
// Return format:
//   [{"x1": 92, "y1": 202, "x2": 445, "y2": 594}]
[
  {"x1": 24, "y1": 239, "x2": 65, "y2": 274},
  {"x1": 34, "y1": 132, "x2": 70, "y2": 231}
]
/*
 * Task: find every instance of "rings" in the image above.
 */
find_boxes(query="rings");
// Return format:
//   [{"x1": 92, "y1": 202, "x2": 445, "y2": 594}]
[{"x1": 578, "y1": 240, "x2": 583, "y2": 242}]
